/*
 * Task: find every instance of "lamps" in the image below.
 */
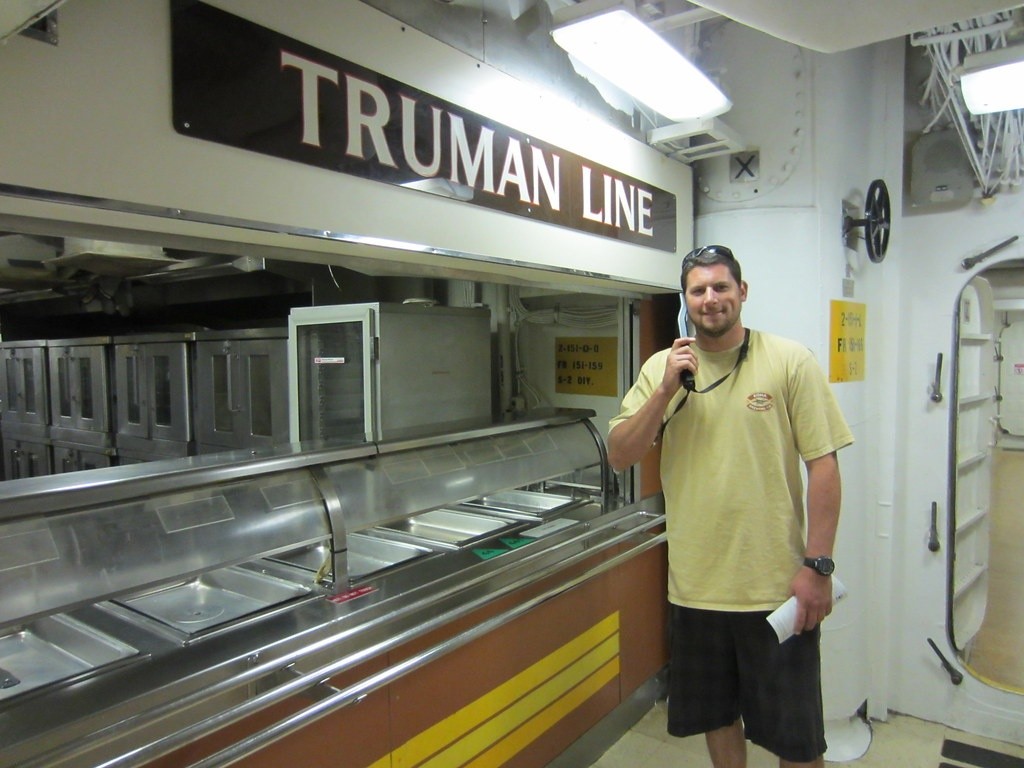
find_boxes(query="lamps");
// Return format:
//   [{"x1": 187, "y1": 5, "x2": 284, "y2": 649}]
[
  {"x1": 547, "y1": 1, "x2": 734, "y2": 122},
  {"x1": 960, "y1": 42, "x2": 1024, "y2": 115}
]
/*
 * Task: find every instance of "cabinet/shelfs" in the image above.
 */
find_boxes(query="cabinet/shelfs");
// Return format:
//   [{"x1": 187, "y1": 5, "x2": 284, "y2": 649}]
[
  {"x1": 0, "y1": 340, "x2": 50, "y2": 480},
  {"x1": 287, "y1": 302, "x2": 492, "y2": 455},
  {"x1": 47, "y1": 336, "x2": 117, "y2": 474},
  {"x1": 114, "y1": 331, "x2": 195, "y2": 467},
  {"x1": 196, "y1": 327, "x2": 290, "y2": 453}
]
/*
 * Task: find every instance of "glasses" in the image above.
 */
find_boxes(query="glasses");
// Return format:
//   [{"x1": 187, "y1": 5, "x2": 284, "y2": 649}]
[{"x1": 682, "y1": 245, "x2": 734, "y2": 268}]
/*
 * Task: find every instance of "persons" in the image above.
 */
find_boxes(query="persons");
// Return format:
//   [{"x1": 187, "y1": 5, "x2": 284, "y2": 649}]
[{"x1": 605, "y1": 245, "x2": 856, "y2": 768}]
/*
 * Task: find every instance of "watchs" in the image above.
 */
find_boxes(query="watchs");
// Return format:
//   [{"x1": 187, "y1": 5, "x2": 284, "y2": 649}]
[{"x1": 803, "y1": 556, "x2": 836, "y2": 575}]
